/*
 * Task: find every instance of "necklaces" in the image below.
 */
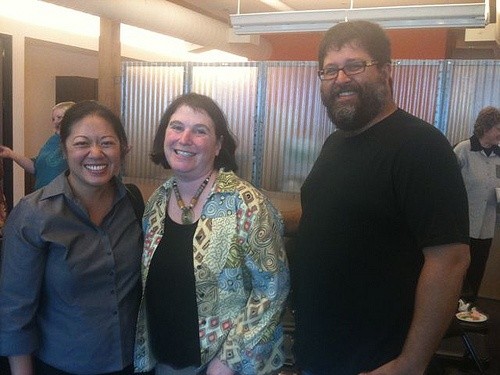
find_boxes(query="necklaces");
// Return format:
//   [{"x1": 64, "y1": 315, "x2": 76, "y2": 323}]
[{"x1": 171, "y1": 171, "x2": 214, "y2": 225}]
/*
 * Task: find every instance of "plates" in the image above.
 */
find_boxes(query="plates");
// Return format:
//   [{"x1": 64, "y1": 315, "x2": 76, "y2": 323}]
[{"x1": 456, "y1": 311, "x2": 488, "y2": 323}]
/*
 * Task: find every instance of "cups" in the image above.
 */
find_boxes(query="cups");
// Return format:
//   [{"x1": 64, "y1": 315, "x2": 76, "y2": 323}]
[{"x1": 495, "y1": 188, "x2": 500, "y2": 203}]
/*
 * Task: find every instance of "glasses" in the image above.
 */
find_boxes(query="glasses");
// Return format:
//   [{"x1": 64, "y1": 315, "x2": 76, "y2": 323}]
[{"x1": 317, "y1": 60, "x2": 377, "y2": 80}]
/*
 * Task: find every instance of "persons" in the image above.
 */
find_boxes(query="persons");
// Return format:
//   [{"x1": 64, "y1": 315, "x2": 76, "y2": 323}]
[
  {"x1": 1, "y1": 101, "x2": 77, "y2": 249},
  {"x1": 453, "y1": 107, "x2": 500, "y2": 319},
  {"x1": 274, "y1": 21, "x2": 471, "y2": 375},
  {"x1": 1, "y1": 100, "x2": 147, "y2": 375},
  {"x1": 141, "y1": 93, "x2": 291, "y2": 375}
]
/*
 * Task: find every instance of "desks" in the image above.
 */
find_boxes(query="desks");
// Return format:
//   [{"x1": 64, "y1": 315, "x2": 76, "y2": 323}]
[{"x1": 459, "y1": 295, "x2": 500, "y2": 375}]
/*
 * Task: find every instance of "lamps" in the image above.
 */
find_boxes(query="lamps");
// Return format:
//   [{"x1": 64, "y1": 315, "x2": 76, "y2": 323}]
[{"x1": 229, "y1": 0, "x2": 492, "y2": 35}]
